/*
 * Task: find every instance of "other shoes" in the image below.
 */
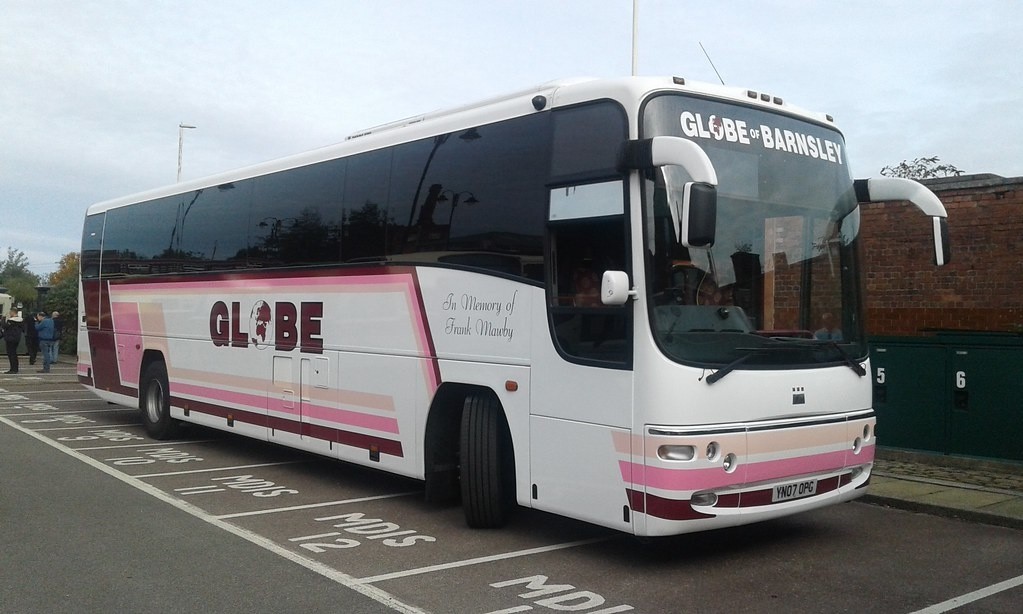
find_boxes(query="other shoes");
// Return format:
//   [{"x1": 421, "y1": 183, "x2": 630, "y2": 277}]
[
  {"x1": 37, "y1": 368, "x2": 50, "y2": 373},
  {"x1": 49, "y1": 362, "x2": 57, "y2": 364},
  {"x1": 4, "y1": 369, "x2": 17, "y2": 374}
]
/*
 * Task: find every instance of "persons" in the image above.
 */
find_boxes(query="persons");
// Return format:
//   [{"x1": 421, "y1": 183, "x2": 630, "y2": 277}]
[
  {"x1": 24, "y1": 312, "x2": 38, "y2": 365},
  {"x1": 34, "y1": 312, "x2": 54, "y2": 373},
  {"x1": 0, "y1": 306, "x2": 23, "y2": 374},
  {"x1": 49, "y1": 311, "x2": 63, "y2": 363}
]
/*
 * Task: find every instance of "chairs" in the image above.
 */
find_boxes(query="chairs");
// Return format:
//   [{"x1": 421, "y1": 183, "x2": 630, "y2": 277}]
[{"x1": 558, "y1": 243, "x2": 731, "y2": 309}]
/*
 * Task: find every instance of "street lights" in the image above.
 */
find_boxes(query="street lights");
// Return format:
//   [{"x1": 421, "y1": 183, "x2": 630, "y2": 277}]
[{"x1": 176, "y1": 120, "x2": 196, "y2": 184}]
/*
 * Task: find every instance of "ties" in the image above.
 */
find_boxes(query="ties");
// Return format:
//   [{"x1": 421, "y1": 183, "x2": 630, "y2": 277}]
[{"x1": 828, "y1": 333, "x2": 832, "y2": 339}]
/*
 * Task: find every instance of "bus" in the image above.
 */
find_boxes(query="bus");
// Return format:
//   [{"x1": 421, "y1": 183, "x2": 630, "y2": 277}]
[{"x1": 75, "y1": 77, "x2": 952, "y2": 539}]
